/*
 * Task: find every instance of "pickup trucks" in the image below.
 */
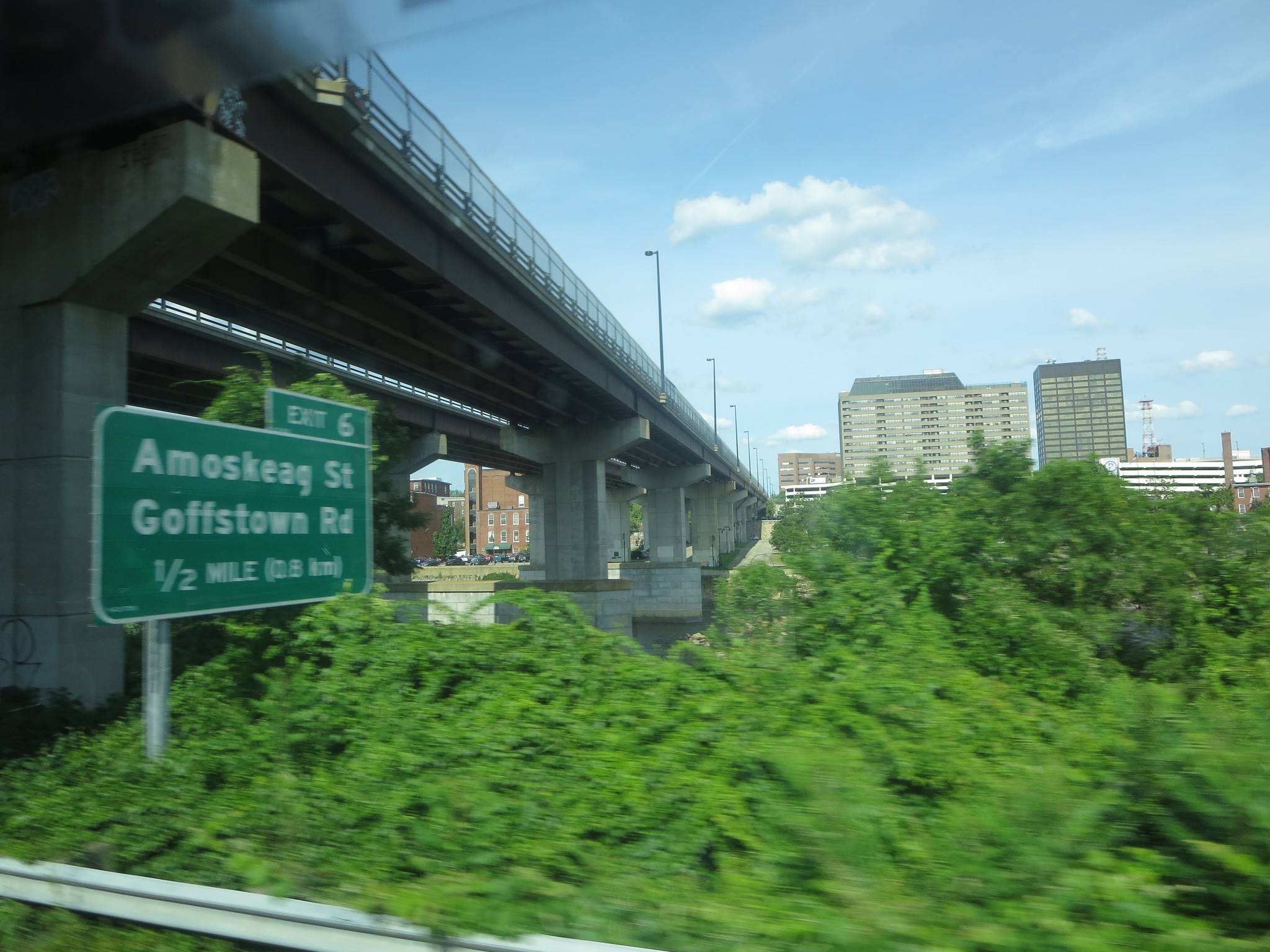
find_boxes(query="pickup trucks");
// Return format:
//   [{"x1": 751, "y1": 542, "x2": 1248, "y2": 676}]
[{"x1": 471, "y1": 554, "x2": 484, "y2": 561}]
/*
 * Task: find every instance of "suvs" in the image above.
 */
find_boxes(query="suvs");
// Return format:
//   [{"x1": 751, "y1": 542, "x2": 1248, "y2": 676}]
[{"x1": 446, "y1": 556, "x2": 465, "y2": 565}]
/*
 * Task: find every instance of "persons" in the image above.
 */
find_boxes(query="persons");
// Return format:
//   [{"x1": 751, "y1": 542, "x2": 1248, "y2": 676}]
[
  {"x1": 637, "y1": 538, "x2": 641, "y2": 547},
  {"x1": 632, "y1": 551, "x2": 642, "y2": 560},
  {"x1": 734, "y1": 538, "x2": 736, "y2": 543}
]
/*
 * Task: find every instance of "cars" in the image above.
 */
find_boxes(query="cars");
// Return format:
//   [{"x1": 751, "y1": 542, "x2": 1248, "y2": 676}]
[
  {"x1": 460, "y1": 556, "x2": 471, "y2": 562},
  {"x1": 748, "y1": 536, "x2": 759, "y2": 541},
  {"x1": 421, "y1": 559, "x2": 440, "y2": 566},
  {"x1": 471, "y1": 556, "x2": 489, "y2": 565},
  {"x1": 414, "y1": 557, "x2": 429, "y2": 564},
  {"x1": 493, "y1": 553, "x2": 530, "y2": 562},
  {"x1": 484, "y1": 555, "x2": 493, "y2": 562}
]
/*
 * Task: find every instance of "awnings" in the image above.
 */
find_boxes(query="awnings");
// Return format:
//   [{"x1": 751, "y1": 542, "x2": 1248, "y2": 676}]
[{"x1": 484, "y1": 543, "x2": 512, "y2": 550}]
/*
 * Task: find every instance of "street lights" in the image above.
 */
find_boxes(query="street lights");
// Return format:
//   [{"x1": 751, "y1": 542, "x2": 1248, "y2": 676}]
[
  {"x1": 718, "y1": 528, "x2": 723, "y2": 560},
  {"x1": 644, "y1": 249, "x2": 665, "y2": 392},
  {"x1": 723, "y1": 526, "x2": 731, "y2": 555},
  {"x1": 733, "y1": 522, "x2": 739, "y2": 546},
  {"x1": 737, "y1": 520, "x2": 743, "y2": 543},
  {"x1": 764, "y1": 469, "x2": 775, "y2": 498},
  {"x1": 753, "y1": 447, "x2": 759, "y2": 487},
  {"x1": 706, "y1": 358, "x2": 717, "y2": 445},
  {"x1": 712, "y1": 534, "x2": 714, "y2": 566},
  {"x1": 744, "y1": 431, "x2": 751, "y2": 479},
  {"x1": 760, "y1": 459, "x2": 765, "y2": 492},
  {"x1": 730, "y1": 405, "x2": 739, "y2": 468}
]
